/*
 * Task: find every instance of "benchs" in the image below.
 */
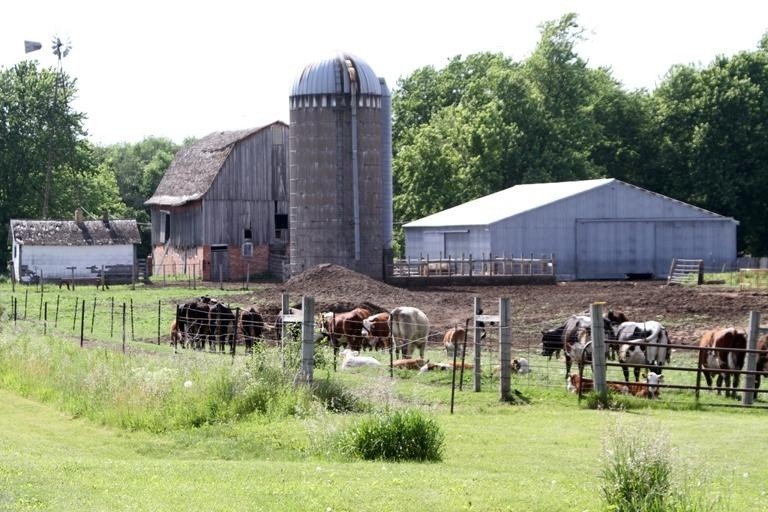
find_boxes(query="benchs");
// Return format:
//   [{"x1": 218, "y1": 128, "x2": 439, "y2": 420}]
[{"x1": 59, "y1": 277, "x2": 109, "y2": 291}]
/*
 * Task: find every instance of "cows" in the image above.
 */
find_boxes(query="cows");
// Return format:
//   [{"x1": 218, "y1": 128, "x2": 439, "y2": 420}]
[
  {"x1": 168, "y1": 295, "x2": 432, "y2": 358},
  {"x1": 338, "y1": 348, "x2": 381, "y2": 370},
  {"x1": 601, "y1": 310, "x2": 673, "y2": 381},
  {"x1": 387, "y1": 358, "x2": 475, "y2": 376},
  {"x1": 493, "y1": 355, "x2": 531, "y2": 376},
  {"x1": 539, "y1": 312, "x2": 611, "y2": 380},
  {"x1": 698, "y1": 326, "x2": 747, "y2": 399},
  {"x1": 565, "y1": 372, "x2": 666, "y2": 401},
  {"x1": 753, "y1": 332, "x2": 768, "y2": 399},
  {"x1": 443, "y1": 326, "x2": 467, "y2": 357}
]
